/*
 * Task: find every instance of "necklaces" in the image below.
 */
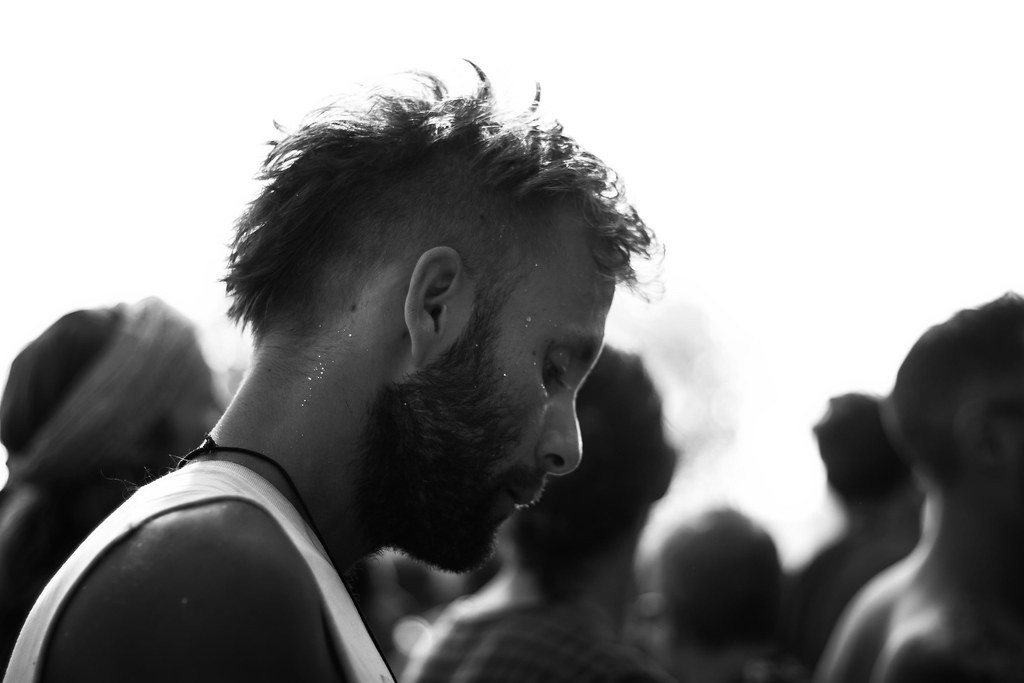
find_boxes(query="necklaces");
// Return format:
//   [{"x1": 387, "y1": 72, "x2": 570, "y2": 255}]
[{"x1": 177, "y1": 433, "x2": 399, "y2": 682}]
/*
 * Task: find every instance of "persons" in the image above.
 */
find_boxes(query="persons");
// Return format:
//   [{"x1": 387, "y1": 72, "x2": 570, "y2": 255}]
[{"x1": 0, "y1": 59, "x2": 1024, "y2": 683}]
